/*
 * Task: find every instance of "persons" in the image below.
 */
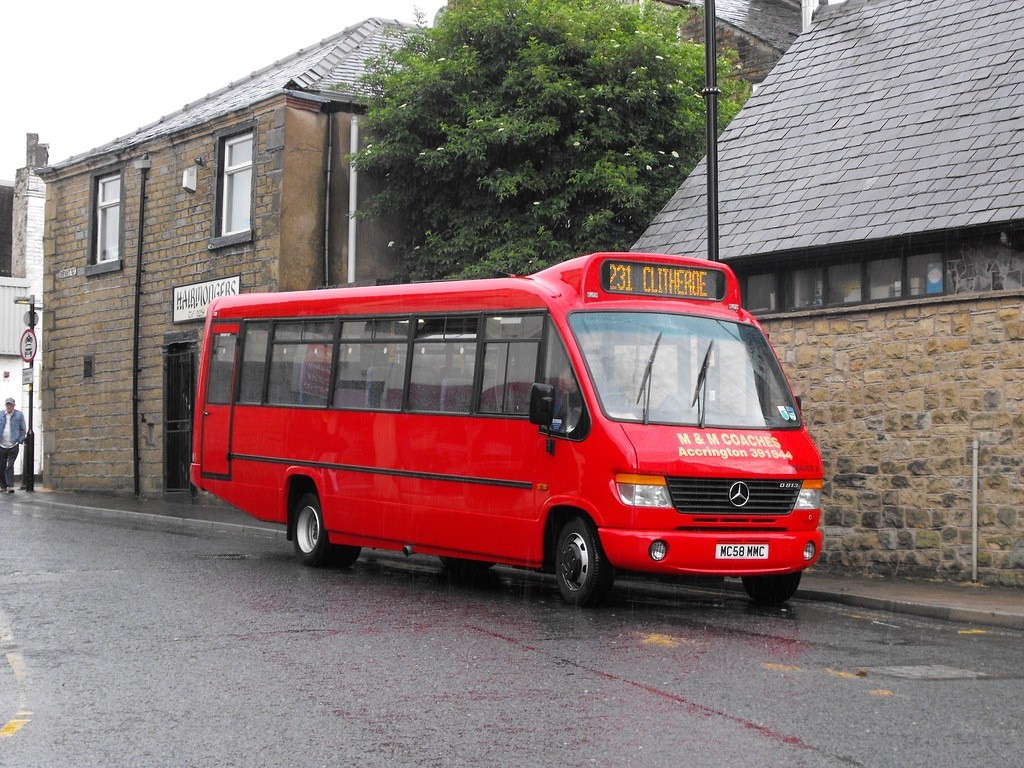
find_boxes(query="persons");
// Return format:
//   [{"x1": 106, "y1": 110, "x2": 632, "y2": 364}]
[
  {"x1": 550, "y1": 361, "x2": 584, "y2": 427},
  {"x1": 0, "y1": 398, "x2": 26, "y2": 493}
]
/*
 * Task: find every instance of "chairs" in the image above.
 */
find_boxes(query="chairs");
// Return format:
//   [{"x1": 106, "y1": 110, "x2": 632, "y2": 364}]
[{"x1": 267, "y1": 360, "x2": 496, "y2": 415}]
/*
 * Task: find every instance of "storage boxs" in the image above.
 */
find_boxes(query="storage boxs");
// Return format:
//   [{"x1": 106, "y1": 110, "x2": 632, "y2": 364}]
[
  {"x1": 894, "y1": 281, "x2": 902, "y2": 296},
  {"x1": 926, "y1": 262, "x2": 943, "y2": 294},
  {"x1": 909, "y1": 278, "x2": 925, "y2": 295}
]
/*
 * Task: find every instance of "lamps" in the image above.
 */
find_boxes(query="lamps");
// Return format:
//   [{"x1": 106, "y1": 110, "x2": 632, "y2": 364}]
[{"x1": 183, "y1": 165, "x2": 197, "y2": 193}]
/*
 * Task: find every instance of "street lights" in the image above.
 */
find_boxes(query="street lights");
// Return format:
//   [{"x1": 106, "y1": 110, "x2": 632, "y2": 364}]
[{"x1": 13, "y1": 295, "x2": 36, "y2": 491}]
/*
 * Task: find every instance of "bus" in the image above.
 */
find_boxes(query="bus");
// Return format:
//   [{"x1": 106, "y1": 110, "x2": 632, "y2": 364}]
[{"x1": 187, "y1": 251, "x2": 829, "y2": 608}]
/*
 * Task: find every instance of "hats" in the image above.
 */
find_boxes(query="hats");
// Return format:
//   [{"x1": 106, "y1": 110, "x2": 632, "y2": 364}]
[{"x1": 5, "y1": 398, "x2": 15, "y2": 405}]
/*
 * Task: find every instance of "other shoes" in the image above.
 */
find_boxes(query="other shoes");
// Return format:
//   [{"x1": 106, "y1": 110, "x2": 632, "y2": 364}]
[
  {"x1": 0, "y1": 487, "x2": 7, "y2": 492},
  {"x1": 8, "y1": 487, "x2": 14, "y2": 493}
]
